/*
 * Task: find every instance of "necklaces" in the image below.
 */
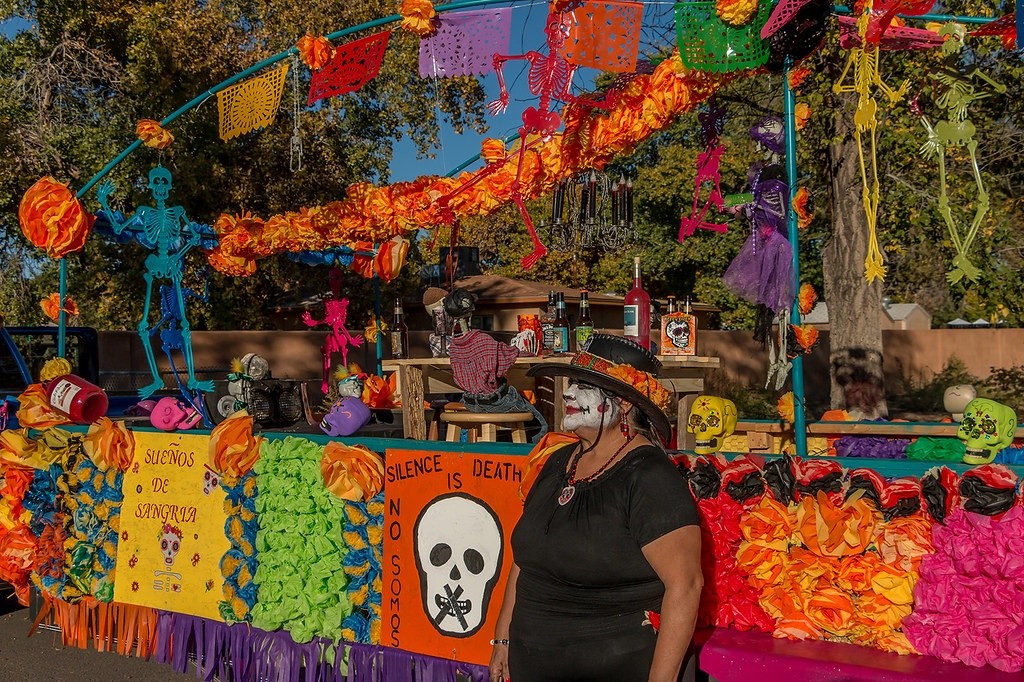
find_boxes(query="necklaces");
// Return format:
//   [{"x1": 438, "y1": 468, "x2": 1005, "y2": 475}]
[{"x1": 557, "y1": 429, "x2": 637, "y2": 506}]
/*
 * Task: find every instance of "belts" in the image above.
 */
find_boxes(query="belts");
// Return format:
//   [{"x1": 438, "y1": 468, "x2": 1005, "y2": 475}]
[{"x1": 464, "y1": 385, "x2": 508, "y2": 404}]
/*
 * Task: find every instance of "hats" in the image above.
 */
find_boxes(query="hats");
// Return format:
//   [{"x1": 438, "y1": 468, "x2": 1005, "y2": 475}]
[
  {"x1": 444, "y1": 288, "x2": 478, "y2": 316},
  {"x1": 526, "y1": 334, "x2": 671, "y2": 445},
  {"x1": 423, "y1": 287, "x2": 449, "y2": 305}
]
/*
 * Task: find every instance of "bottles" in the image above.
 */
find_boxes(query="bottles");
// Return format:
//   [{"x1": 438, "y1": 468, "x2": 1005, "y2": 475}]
[
  {"x1": 667, "y1": 295, "x2": 676, "y2": 314},
  {"x1": 624, "y1": 256, "x2": 651, "y2": 352},
  {"x1": 390, "y1": 297, "x2": 409, "y2": 360},
  {"x1": 682, "y1": 295, "x2": 693, "y2": 315},
  {"x1": 553, "y1": 292, "x2": 570, "y2": 353},
  {"x1": 576, "y1": 290, "x2": 594, "y2": 354},
  {"x1": 542, "y1": 290, "x2": 557, "y2": 355}
]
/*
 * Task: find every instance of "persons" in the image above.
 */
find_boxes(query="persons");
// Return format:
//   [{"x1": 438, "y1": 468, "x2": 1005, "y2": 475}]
[{"x1": 488, "y1": 333, "x2": 706, "y2": 682}]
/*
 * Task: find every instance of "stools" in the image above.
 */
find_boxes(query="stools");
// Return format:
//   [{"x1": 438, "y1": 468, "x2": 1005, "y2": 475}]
[{"x1": 441, "y1": 413, "x2": 533, "y2": 444}]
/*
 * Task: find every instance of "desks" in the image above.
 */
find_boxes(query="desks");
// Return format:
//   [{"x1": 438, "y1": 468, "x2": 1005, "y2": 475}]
[{"x1": 382, "y1": 356, "x2": 720, "y2": 461}]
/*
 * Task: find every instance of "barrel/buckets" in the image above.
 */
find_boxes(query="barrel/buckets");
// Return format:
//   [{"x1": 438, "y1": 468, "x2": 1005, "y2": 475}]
[{"x1": 516, "y1": 313, "x2": 543, "y2": 356}]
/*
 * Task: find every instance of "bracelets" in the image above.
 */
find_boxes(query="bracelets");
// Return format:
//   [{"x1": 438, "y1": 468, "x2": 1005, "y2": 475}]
[{"x1": 489, "y1": 638, "x2": 509, "y2": 646}]
